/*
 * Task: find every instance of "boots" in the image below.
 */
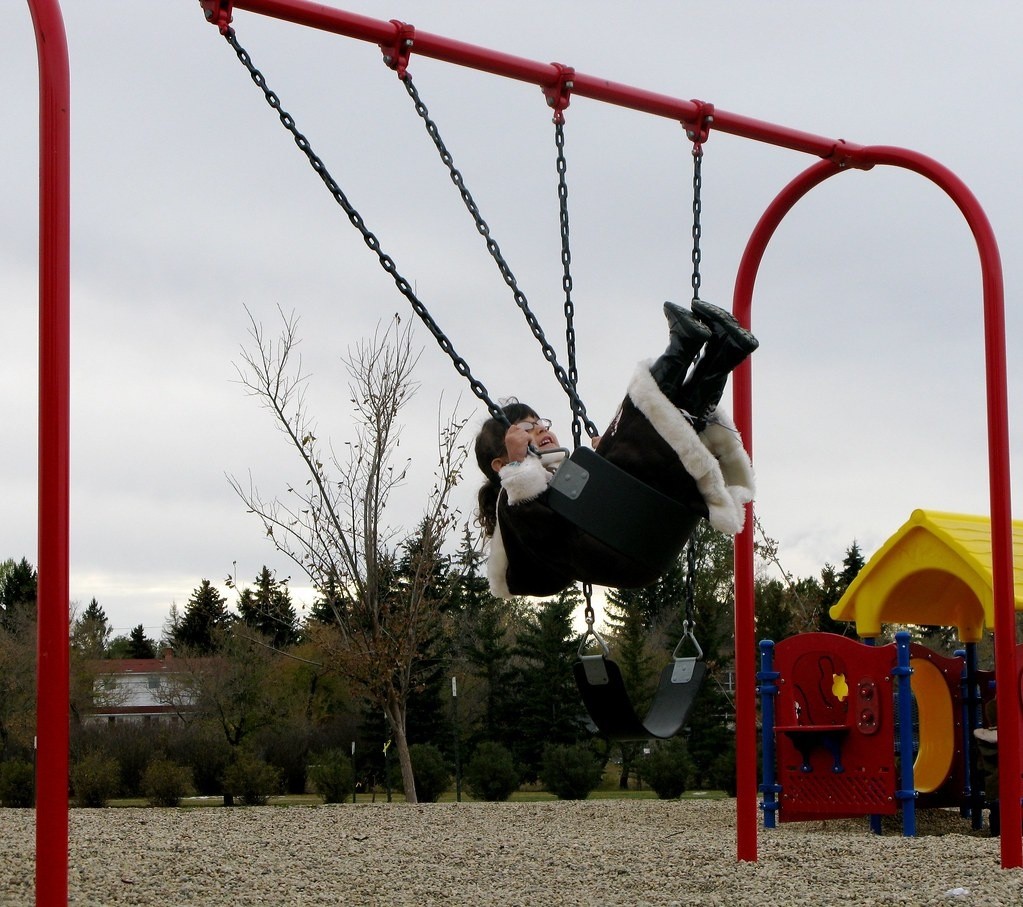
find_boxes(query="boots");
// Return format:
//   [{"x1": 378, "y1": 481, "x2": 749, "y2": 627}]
[
  {"x1": 650, "y1": 301, "x2": 712, "y2": 407},
  {"x1": 675, "y1": 299, "x2": 759, "y2": 436}
]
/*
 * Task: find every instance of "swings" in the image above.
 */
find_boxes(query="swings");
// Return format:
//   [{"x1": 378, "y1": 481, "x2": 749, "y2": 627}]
[
  {"x1": 218, "y1": 33, "x2": 696, "y2": 571},
  {"x1": 552, "y1": 111, "x2": 712, "y2": 742}
]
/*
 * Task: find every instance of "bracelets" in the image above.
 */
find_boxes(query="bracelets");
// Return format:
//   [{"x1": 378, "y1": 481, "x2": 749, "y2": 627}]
[{"x1": 509, "y1": 462, "x2": 520, "y2": 466}]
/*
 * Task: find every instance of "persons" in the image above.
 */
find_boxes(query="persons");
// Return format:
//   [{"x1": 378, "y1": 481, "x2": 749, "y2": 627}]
[{"x1": 475, "y1": 300, "x2": 758, "y2": 596}]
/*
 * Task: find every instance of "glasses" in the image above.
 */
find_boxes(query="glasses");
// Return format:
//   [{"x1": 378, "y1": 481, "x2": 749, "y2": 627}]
[{"x1": 517, "y1": 418, "x2": 552, "y2": 431}]
